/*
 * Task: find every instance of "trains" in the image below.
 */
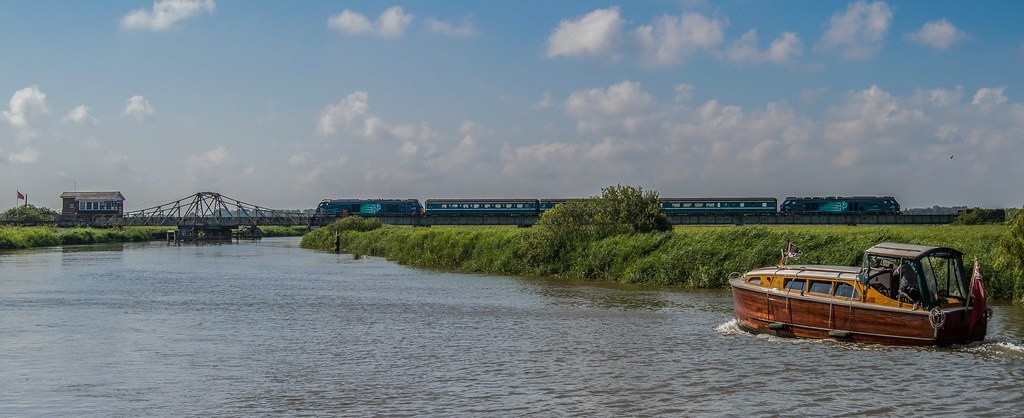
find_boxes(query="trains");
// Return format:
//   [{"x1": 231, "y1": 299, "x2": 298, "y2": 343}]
[{"x1": 315, "y1": 195, "x2": 900, "y2": 216}]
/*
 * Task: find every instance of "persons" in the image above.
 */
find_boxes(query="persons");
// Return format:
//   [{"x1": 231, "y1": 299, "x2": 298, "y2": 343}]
[{"x1": 893, "y1": 261, "x2": 920, "y2": 303}]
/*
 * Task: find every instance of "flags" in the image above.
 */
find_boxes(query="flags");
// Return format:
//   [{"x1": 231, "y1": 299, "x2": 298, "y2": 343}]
[
  {"x1": 785, "y1": 242, "x2": 802, "y2": 258},
  {"x1": 969, "y1": 262, "x2": 986, "y2": 330}
]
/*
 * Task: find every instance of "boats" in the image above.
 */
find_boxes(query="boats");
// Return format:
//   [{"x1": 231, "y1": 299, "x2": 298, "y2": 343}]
[
  {"x1": 242, "y1": 227, "x2": 263, "y2": 238},
  {"x1": 729, "y1": 240, "x2": 991, "y2": 346}
]
